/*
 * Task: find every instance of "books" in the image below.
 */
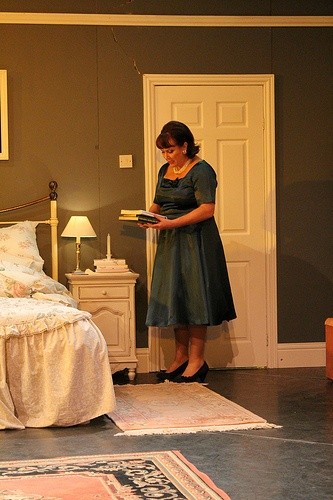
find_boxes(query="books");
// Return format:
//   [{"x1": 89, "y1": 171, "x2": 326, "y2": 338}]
[
  {"x1": 93, "y1": 259, "x2": 130, "y2": 273},
  {"x1": 118, "y1": 208, "x2": 168, "y2": 224}
]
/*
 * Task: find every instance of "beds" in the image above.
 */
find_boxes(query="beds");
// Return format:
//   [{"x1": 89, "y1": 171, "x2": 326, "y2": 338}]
[{"x1": 0, "y1": 180, "x2": 116, "y2": 430}]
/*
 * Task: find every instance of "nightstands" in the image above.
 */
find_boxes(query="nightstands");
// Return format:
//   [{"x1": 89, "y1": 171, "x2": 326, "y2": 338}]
[{"x1": 65, "y1": 273, "x2": 140, "y2": 380}]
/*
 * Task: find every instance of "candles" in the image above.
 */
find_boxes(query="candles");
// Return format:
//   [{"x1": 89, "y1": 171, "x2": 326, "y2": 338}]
[{"x1": 106, "y1": 233, "x2": 111, "y2": 255}]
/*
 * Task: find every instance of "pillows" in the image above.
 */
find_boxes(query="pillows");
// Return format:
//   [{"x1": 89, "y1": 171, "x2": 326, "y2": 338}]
[{"x1": 0, "y1": 220, "x2": 44, "y2": 273}]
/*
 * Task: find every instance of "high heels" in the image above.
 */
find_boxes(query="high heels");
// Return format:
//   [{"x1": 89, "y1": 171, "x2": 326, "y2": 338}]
[
  {"x1": 155, "y1": 359, "x2": 189, "y2": 383},
  {"x1": 173, "y1": 361, "x2": 209, "y2": 383}
]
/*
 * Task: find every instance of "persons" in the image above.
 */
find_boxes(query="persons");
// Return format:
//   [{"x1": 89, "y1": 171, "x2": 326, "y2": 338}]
[{"x1": 145, "y1": 119, "x2": 237, "y2": 383}]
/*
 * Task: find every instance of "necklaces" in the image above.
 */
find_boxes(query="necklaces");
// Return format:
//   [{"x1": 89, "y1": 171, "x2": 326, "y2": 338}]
[{"x1": 173, "y1": 153, "x2": 197, "y2": 174}]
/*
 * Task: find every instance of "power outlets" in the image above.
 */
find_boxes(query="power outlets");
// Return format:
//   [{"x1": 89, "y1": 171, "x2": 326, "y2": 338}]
[{"x1": 119, "y1": 155, "x2": 133, "y2": 168}]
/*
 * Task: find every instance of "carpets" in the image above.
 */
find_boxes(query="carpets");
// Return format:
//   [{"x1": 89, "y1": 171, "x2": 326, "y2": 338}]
[
  {"x1": 0, "y1": 450, "x2": 231, "y2": 500},
  {"x1": 105, "y1": 379, "x2": 283, "y2": 436}
]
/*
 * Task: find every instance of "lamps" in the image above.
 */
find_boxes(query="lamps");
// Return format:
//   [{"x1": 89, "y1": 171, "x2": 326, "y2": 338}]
[{"x1": 60, "y1": 216, "x2": 97, "y2": 273}]
